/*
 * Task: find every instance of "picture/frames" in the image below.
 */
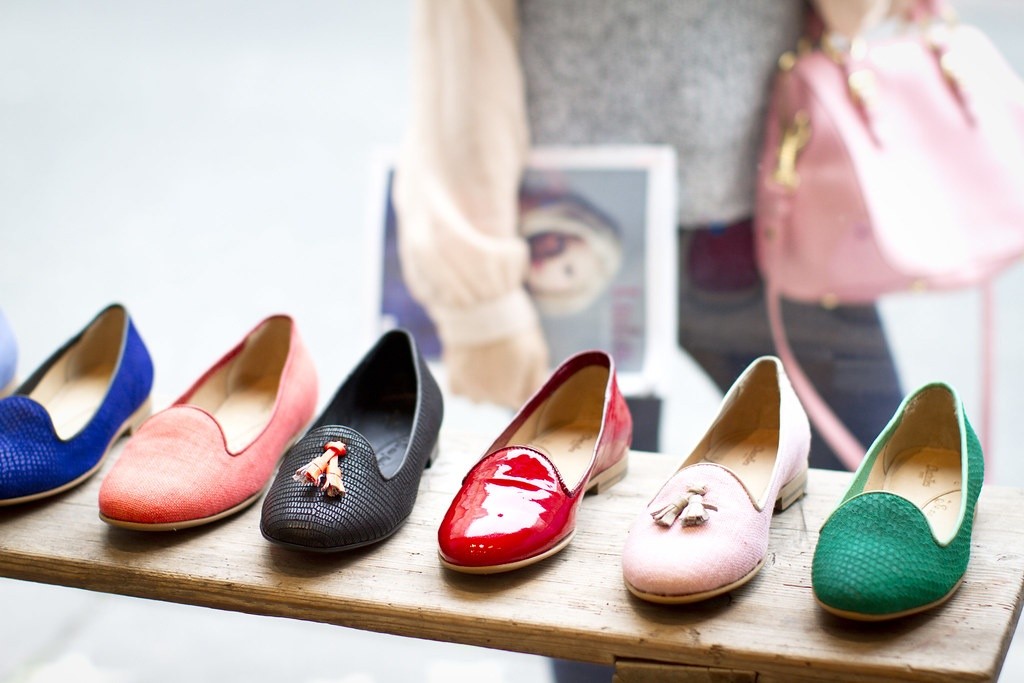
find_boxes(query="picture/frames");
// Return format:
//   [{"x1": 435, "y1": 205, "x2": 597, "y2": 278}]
[{"x1": 350, "y1": 141, "x2": 675, "y2": 397}]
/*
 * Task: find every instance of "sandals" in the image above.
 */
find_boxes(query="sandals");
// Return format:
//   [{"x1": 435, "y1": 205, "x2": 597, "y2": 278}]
[{"x1": 810, "y1": 381, "x2": 985, "y2": 622}]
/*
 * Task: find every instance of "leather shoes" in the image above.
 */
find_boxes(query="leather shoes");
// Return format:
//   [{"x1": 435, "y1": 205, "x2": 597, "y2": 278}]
[{"x1": 436, "y1": 350, "x2": 632, "y2": 576}]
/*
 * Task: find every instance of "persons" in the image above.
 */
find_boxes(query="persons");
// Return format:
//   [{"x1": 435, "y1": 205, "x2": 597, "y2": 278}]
[
  {"x1": 381, "y1": 192, "x2": 622, "y2": 369},
  {"x1": 393, "y1": 0, "x2": 1024, "y2": 683}
]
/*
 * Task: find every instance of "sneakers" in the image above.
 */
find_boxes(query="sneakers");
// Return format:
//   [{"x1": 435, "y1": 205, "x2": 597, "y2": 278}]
[
  {"x1": 96, "y1": 313, "x2": 319, "y2": 532},
  {"x1": 620, "y1": 354, "x2": 812, "y2": 604},
  {"x1": 0, "y1": 303, "x2": 156, "y2": 511},
  {"x1": 259, "y1": 328, "x2": 446, "y2": 555},
  {"x1": 751, "y1": 0, "x2": 1023, "y2": 475}
]
